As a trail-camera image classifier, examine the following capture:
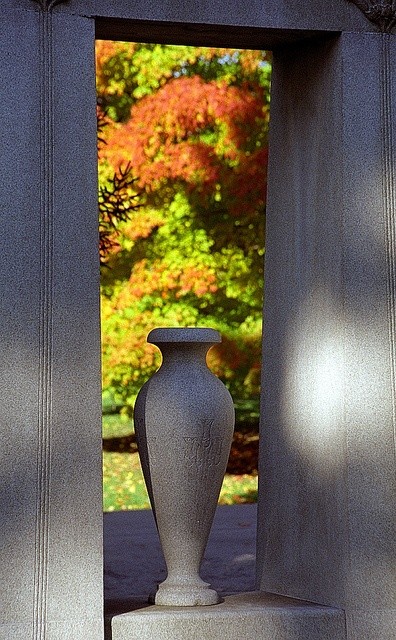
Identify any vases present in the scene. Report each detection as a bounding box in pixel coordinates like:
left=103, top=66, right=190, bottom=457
left=134, top=327, right=236, bottom=608
